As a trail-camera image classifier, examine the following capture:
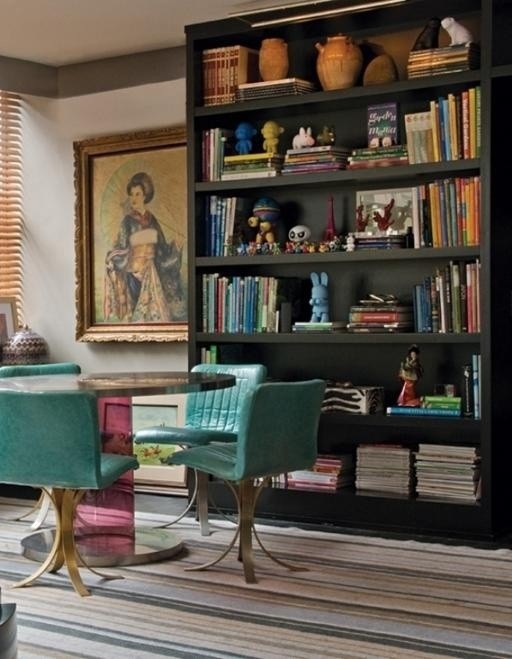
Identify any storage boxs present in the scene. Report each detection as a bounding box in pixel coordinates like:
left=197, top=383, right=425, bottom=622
left=320, top=379, right=387, bottom=417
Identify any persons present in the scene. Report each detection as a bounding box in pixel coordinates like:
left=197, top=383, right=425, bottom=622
left=396, top=345, right=424, bottom=407
left=98, top=171, right=183, bottom=326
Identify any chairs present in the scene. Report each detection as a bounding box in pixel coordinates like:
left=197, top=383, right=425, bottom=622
left=129, top=360, right=269, bottom=543
left=163, top=373, right=331, bottom=585
left=0, top=360, right=98, bottom=530
left=0, top=386, right=128, bottom=600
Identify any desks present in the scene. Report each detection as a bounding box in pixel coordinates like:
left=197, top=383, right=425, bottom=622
left=0, top=370, right=238, bottom=570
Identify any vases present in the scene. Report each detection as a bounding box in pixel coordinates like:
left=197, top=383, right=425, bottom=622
left=258, top=38, right=290, bottom=82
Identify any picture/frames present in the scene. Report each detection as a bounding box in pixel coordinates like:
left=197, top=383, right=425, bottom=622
left=95, top=393, right=188, bottom=489
left=0, top=295, right=22, bottom=354
left=65, top=120, right=187, bottom=345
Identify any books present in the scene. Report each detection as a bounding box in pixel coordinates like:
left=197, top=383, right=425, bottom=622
left=203, top=260, right=481, bottom=333
left=386, top=354, right=481, bottom=420
left=202, top=87, right=483, bottom=182
left=202, top=45, right=314, bottom=106
left=406, top=44, right=480, bottom=78
left=253, top=443, right=481, bottom=501
left=412, top=176, right=483, bottom=248
left=204, top=195, right=253, bottom=256
left=201, top=344, right=239, bottom=364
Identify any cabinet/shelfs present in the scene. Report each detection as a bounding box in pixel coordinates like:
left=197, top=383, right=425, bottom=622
left=183, top=0, right=511, bottom=550
